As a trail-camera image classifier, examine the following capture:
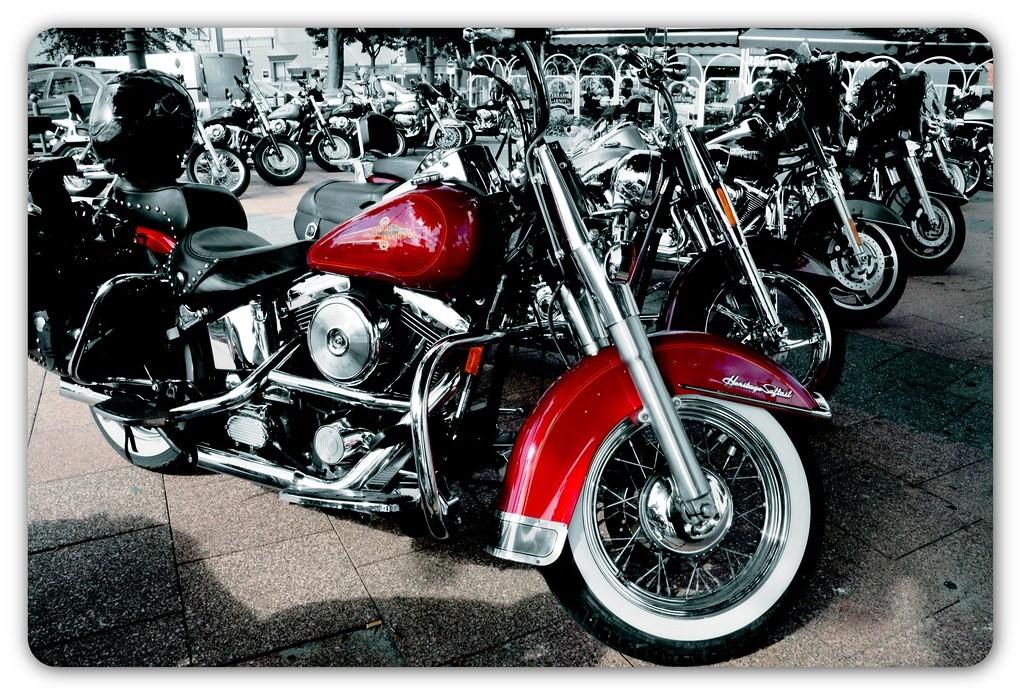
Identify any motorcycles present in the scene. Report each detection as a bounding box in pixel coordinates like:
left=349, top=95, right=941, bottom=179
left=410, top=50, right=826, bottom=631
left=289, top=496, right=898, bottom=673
left=27, top=27, right=994, bottom=669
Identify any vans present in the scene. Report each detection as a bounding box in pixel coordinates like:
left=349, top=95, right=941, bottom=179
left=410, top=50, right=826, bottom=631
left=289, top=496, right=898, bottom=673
left=61, top=54, right=250, bottom=122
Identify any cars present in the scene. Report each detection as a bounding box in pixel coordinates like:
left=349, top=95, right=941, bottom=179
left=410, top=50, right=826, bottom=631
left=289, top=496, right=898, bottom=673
left=29, top=67, right=122, bottom=121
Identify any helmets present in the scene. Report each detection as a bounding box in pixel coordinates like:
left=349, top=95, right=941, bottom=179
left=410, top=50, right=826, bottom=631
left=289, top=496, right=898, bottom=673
left=88, top=67, right=199, bottom=182
